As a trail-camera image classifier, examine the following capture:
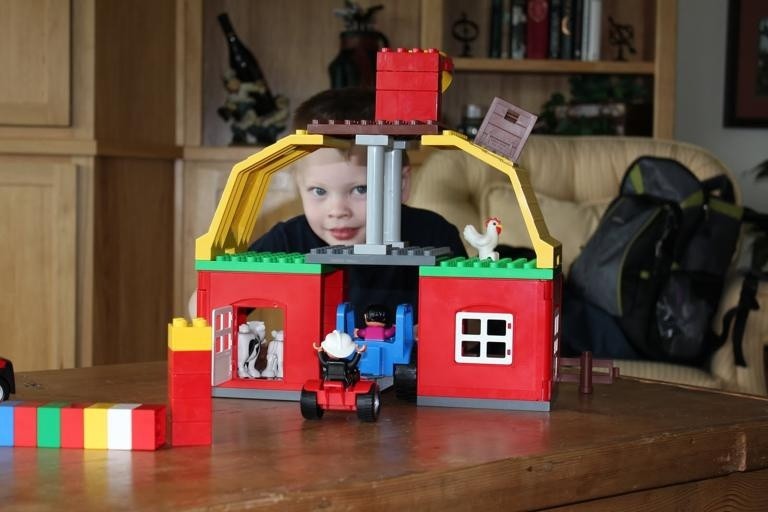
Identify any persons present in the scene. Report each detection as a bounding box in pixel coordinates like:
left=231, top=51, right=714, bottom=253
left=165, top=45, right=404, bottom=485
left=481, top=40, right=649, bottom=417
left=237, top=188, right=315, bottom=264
left=247, top=86, right=470, bottom=330
left=354, top=305, right=395, bottom=341
left=313, top=330, right=366, bottom=372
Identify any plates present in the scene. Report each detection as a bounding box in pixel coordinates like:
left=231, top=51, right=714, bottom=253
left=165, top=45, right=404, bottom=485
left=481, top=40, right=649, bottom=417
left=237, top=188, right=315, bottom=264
left=167, top=3, right=686, bottom=333
left=0, top=2, right=183, bottom=375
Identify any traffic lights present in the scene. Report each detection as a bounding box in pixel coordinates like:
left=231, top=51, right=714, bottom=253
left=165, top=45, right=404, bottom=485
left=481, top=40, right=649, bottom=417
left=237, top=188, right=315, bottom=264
left=465, top=180, right=618, bottom=287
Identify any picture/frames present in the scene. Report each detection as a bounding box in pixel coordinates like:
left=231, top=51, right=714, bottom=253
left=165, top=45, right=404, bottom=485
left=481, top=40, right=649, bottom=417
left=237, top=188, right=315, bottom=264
left=0, top=352, right=767, bottom=511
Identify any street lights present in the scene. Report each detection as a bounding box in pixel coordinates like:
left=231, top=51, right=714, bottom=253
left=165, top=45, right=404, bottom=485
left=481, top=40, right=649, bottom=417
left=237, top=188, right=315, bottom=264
left=559, top=155, right=767, bottom=370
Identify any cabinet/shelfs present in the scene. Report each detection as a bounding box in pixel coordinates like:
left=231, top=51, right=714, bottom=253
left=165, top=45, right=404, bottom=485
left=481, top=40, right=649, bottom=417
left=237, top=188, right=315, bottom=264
left=214, top=12, right=277, bottom=118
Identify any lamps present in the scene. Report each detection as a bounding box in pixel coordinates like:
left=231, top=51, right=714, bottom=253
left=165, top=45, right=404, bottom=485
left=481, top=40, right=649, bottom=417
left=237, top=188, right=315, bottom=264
left=397, top=132, right=766, bottom=401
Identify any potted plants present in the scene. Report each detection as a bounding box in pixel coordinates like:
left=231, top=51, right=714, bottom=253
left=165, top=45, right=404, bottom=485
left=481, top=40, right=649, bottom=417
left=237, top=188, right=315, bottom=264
left=722, top=0, right=767, bottom=133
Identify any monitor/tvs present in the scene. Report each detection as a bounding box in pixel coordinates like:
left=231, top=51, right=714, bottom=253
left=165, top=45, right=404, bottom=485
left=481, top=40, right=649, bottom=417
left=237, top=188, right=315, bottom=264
left=489, top=2, right=603, bottom=62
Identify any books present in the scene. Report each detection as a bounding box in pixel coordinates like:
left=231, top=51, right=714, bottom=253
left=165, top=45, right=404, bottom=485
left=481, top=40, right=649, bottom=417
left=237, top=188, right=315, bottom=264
left=489, top=2, right=603, bottom=62
